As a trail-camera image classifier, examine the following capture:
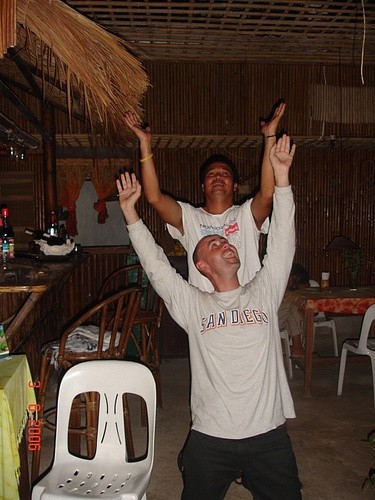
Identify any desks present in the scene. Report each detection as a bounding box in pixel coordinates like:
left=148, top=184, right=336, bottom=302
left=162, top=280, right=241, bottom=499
left=0, top=251, right=90, bottom=354
left=0, top=355, right=39, bottom=500
left=283, top=284, right=375, bottom=397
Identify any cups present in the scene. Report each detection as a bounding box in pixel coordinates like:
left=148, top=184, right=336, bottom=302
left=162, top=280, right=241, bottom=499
left=320, top=279, right=331, bottom=292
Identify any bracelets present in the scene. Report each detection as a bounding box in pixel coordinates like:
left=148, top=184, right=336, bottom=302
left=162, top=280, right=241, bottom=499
left=140, top=153, right=152, bottom=162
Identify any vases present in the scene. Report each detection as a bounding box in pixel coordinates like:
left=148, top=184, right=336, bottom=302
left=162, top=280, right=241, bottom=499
left=349, top=268, right=358, bottom=289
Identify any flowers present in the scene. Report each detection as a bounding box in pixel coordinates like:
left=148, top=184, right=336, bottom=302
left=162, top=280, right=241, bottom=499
left=338, top=242, right=372, bottom=273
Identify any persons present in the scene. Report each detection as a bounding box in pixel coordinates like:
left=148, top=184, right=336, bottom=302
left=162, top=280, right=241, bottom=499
left=124, top=104, right=287, bottom=294
left=116, top=134, right=304, bottom=500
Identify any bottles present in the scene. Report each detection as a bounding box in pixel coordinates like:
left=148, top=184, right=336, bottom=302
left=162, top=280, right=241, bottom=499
left=48, top=211, right=59, bottom=237
left=0, top=208, right=15, bottom=264
left=24, top=228, right=50, bottom=241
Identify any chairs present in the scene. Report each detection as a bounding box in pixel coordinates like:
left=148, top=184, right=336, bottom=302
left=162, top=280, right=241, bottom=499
left=278, top=278, right=375, bottom=396
left=27, top=265, right=167, bottom=500
